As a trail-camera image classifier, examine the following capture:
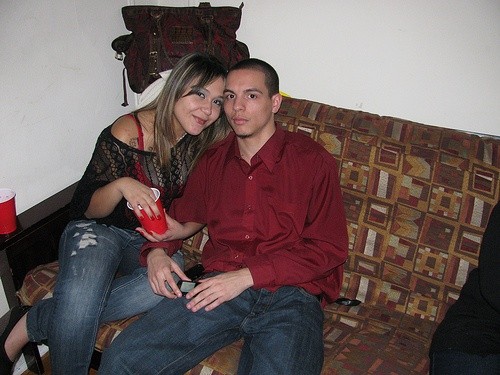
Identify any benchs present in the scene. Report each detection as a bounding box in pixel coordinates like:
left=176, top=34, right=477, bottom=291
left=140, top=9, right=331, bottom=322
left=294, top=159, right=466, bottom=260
left=1, top=94, right=499, bottom=375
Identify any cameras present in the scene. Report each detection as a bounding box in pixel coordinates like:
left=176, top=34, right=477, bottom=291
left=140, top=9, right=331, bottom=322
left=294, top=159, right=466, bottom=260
left=173, top=280, right=203, bottom=297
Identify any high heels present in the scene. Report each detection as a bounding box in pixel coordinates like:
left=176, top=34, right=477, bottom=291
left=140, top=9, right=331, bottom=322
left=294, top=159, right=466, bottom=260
left=0, top=304, right=31, bottom=375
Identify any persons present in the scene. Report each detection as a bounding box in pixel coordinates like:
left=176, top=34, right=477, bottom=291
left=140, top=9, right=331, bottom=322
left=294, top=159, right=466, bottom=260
left=429, top=198, right=500, bottom=375
left=95, top=59, right=349, bottom=375
left=1, top=52, right=234, bottom=375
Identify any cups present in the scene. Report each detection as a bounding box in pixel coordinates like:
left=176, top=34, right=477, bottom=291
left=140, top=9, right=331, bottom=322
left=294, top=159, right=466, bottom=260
left=127, top=188, right=168, bottom=235
left=0, top=188, right=16, bottom=234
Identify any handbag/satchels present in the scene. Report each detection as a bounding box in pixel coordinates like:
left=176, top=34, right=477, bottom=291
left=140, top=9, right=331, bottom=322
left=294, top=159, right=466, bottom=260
left=111, top=2, right=250, bottom=106
